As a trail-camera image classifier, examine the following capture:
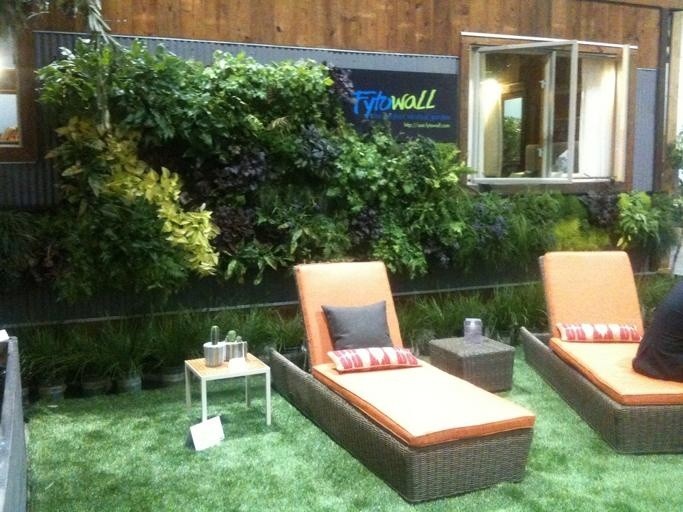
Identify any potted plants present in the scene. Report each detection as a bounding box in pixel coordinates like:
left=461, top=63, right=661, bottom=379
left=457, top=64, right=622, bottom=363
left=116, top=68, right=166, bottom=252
left=157, top=303, right=201, bottom=385
left=203, top=326, right=223, bottom=367
left=70, top=324, right=111, bottom=397
left=19, top=320, right=70, bottom=402
left=112, top=306, right=163, bottom=397
left=225, top=330, right=247, bottom=361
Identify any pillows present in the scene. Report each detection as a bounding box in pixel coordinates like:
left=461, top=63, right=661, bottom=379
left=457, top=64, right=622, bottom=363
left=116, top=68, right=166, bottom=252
left=321, top=300, right=394, bottom=351
left=324, top=346, right=422, bottom=375
left=554, top=320, right=643, bottom=342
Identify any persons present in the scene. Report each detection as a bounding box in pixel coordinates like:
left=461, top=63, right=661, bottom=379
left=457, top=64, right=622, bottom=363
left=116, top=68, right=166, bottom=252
left=634, top=271, right=679, bottom=379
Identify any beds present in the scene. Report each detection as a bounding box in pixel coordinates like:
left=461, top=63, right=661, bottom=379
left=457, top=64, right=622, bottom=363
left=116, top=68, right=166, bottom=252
left=269, top=261, right=536, bottom=503
left=519, top=251, right=682, bottom=454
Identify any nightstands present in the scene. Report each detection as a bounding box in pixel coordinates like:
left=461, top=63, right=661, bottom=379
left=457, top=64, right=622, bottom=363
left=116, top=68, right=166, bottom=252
left=429, top=336, right=515, bottom=393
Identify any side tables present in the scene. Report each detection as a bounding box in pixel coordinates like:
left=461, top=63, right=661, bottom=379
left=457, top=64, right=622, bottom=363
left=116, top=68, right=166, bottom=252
left=185, top=353, right=272, bottom=425
